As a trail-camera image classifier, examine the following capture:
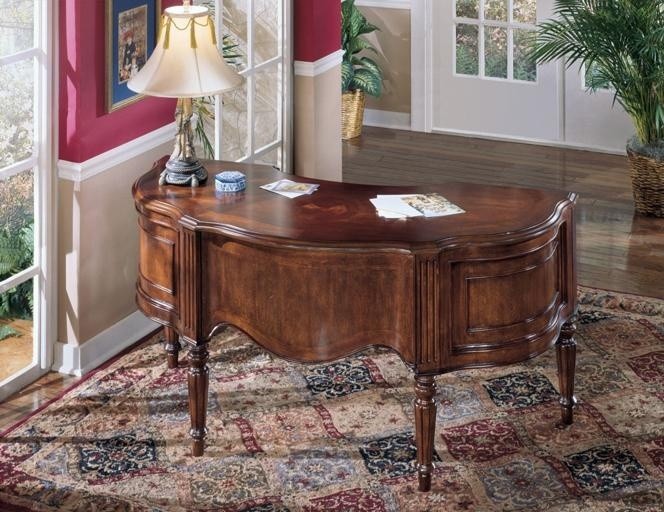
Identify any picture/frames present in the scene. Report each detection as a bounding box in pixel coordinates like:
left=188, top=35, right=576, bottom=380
left=103, top=0, right=162, bottom=116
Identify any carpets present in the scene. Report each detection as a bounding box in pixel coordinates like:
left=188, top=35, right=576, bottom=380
left=0, top=284, right=664, bottom=512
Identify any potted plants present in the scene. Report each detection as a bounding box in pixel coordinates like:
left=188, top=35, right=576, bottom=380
left=341, top=1, right=385, bottom=142
left=519, top=1, right=663, bottom=220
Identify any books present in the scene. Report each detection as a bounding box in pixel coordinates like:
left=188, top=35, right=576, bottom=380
left=256, top=175, right=321, bottom=202
left=368, top=190, right=466, bottom=223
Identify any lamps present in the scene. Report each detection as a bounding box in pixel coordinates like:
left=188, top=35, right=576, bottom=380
left=126, top=4, right=249, bottom=191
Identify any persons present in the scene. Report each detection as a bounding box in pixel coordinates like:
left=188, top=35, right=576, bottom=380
left=121, top=27, right=139, bottom=71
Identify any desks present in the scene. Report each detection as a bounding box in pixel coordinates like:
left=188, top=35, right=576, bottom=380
left=131, top=153, right=579, bottom=495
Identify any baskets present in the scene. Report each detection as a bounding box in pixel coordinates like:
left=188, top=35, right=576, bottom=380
left=342, top=89, right=365, bottom=141
left=627, top=136, right=663, bottom=216
